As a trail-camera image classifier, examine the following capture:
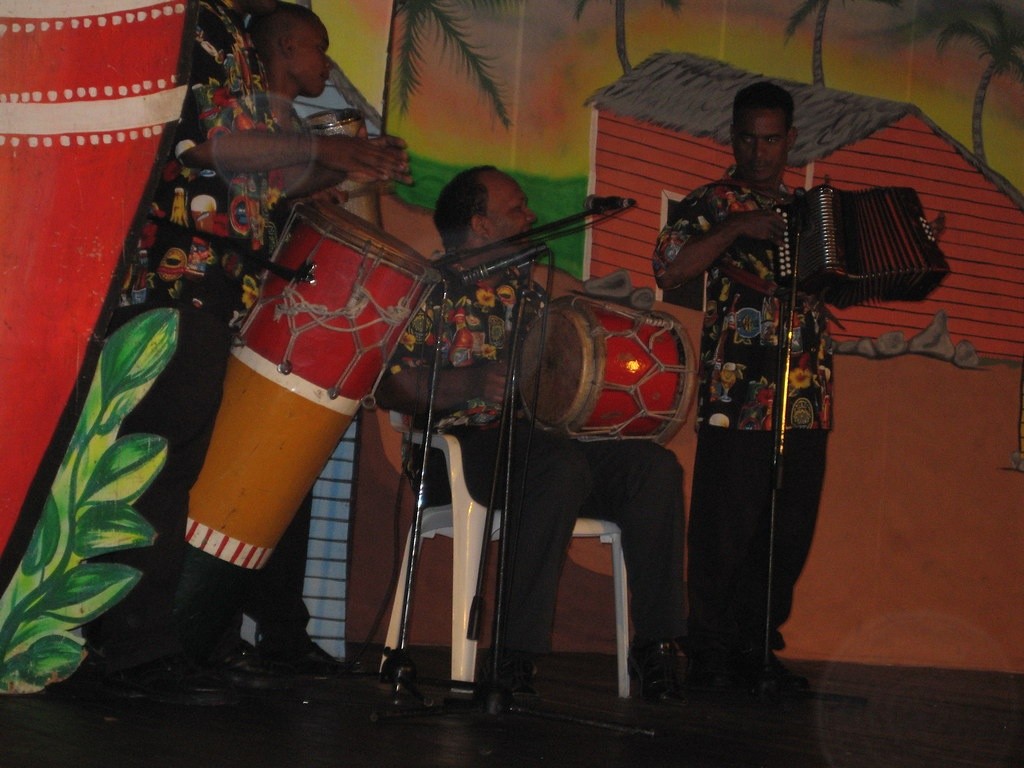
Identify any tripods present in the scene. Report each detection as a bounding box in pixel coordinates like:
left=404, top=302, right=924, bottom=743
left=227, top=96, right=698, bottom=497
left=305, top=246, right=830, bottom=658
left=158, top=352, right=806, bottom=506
left=369, top=248, right=656, bottom=740
left=321, top=210, right=606, bottom=709
left=681, top=187, right=869, bottom=713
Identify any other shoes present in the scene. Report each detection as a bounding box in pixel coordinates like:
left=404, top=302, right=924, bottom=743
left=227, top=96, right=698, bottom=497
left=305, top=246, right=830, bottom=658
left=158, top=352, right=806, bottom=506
left=256, top=635, right=336, bottom=675
left=688, top=657, right=778, bottom=705
left=626, top=635, right=684, bottom=701
left=487, top=644, right=537, bottom=702
left=198, top=643, right=294, bottom=689
left=726, top=650, right=810, bottom=693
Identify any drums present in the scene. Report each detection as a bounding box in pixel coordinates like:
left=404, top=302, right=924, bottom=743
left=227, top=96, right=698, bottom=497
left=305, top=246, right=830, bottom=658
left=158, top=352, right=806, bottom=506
left=515, top=289, right=701, bottom=447
left=183, top=189, right=443, bottom=575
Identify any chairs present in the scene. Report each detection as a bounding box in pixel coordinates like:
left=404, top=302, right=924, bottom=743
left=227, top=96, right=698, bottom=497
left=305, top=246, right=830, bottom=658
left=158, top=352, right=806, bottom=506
left=379, top=410, right=631, bottom=701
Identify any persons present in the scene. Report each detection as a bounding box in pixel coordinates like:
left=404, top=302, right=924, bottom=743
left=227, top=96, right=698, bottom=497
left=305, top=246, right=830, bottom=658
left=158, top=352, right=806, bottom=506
left=652, top=81, right=946, bottom=703
left=80, top=2, right=414, bottom=704
left=249, top=2, right=361, bottom=681
left=375, top=163, right=690, bottom=700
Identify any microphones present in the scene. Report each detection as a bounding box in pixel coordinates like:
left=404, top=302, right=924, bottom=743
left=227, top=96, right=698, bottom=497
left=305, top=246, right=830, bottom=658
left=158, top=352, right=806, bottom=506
left=583, top=194, right=636, bottom=210
left=457, top=241, right=548, bottom=287
